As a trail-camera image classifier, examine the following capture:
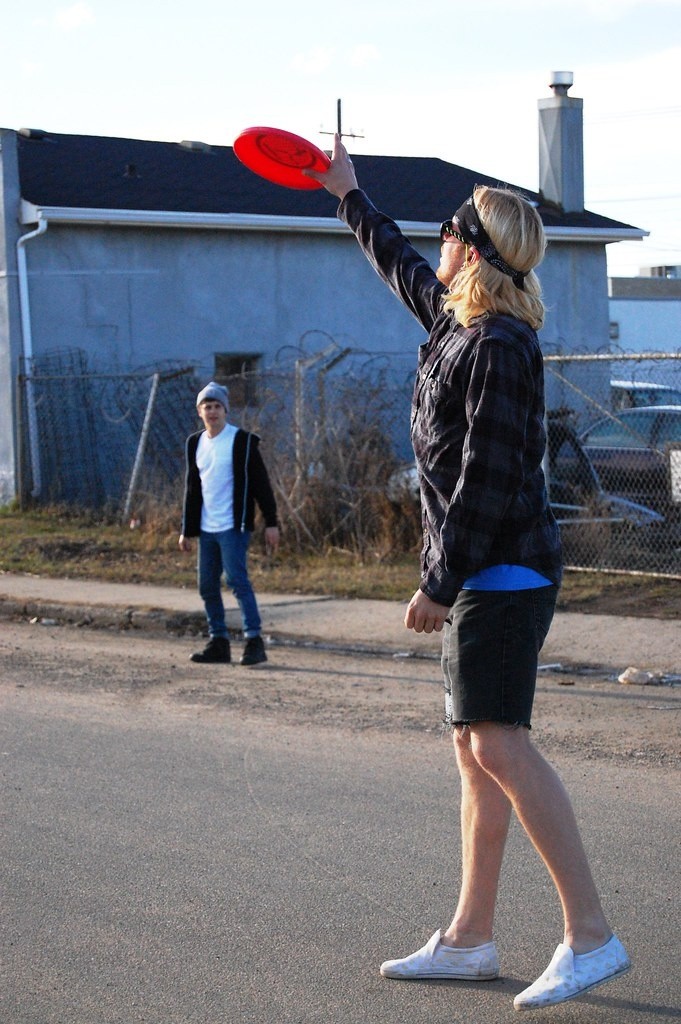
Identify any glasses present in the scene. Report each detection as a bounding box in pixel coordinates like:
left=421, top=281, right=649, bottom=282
left=438, top=219, right=472, bottom=248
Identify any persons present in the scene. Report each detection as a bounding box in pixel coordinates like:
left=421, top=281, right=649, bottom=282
left=302, top=133, right=633, bottom=1011
left=179, top=382, right=279, bottom=666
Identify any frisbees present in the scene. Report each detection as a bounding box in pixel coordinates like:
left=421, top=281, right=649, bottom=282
left=233, top=126, right=331, bottom=191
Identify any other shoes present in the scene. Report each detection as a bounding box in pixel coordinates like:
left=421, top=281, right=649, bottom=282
left=512, top=933, right=634, bottom=1012
left=190, top=638, right=230, bottom=662
left=379, top=926, right=499, bottom=980
left=240, top=637, right=268, bottom=664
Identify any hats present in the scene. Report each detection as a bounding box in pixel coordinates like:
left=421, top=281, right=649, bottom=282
left=197, top=381, right=231, bottom=413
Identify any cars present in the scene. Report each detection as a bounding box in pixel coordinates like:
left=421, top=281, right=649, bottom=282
left=556, top=406, right=681, bottom=502
